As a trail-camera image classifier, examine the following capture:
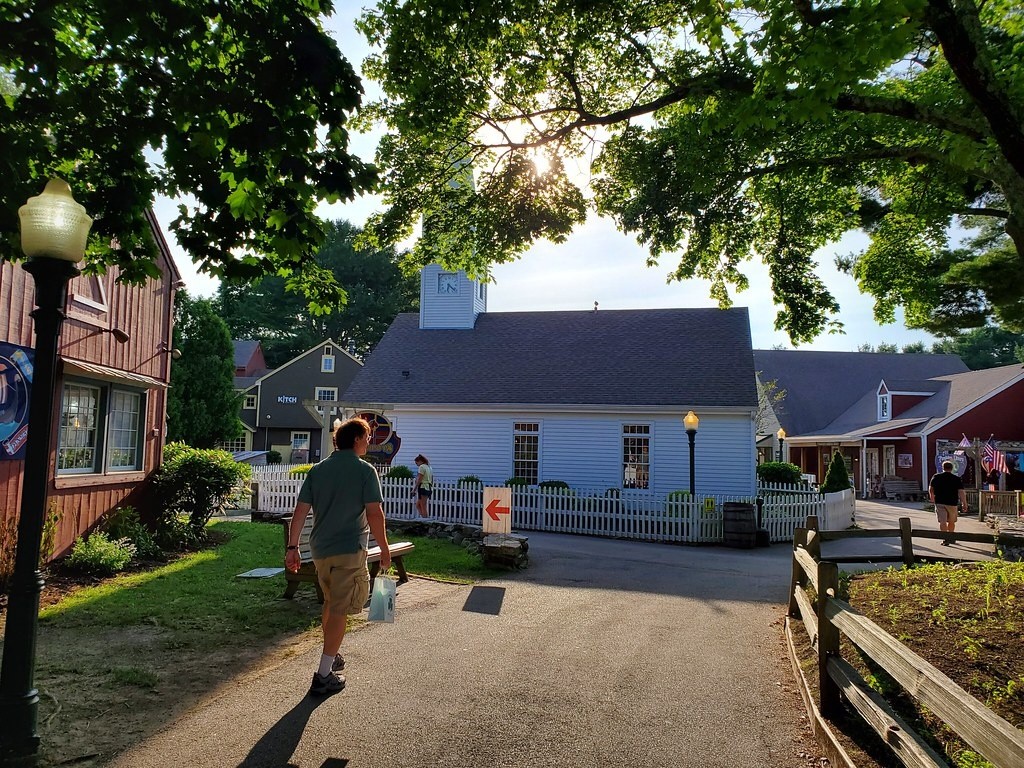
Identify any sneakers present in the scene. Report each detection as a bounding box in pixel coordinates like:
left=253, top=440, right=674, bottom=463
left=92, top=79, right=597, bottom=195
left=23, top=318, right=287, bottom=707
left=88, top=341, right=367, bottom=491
left=332, top=653, right=345, bottom=671
left=310, top=670, right=346, bottom=695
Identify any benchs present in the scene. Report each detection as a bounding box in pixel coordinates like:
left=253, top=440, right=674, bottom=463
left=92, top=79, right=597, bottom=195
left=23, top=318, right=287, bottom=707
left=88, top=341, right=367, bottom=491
left=281, top=515, right=415, bottom=604
left=874, top=479, right=928, bottom=501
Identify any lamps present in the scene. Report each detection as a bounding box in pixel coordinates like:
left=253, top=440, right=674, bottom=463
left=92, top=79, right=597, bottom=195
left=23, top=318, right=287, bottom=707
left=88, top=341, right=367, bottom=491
left=162, top=347, right=182, bottom=358
left=402, top=371, right=409, bottom=379
left=99, top=327, right=130, bottom=344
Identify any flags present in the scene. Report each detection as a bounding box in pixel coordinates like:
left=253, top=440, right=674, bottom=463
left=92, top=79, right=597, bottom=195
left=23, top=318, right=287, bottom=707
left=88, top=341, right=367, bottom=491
left=954, top=437, right=971, bottom=455
left=984, top=436, right=1010, bottom=474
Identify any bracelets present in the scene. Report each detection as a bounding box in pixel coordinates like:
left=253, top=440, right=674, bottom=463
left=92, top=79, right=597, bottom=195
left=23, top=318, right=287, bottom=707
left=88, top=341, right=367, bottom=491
left=286, top=545, right=299, bottom=550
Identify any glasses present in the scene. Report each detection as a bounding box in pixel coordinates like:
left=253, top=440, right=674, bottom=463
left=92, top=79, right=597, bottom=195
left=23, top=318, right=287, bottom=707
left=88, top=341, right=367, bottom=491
left=362, top=436, right=371, bottom=442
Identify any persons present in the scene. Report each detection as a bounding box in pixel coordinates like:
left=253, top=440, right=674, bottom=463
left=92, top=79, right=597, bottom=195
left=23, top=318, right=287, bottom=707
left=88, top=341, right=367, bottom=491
left=986, top=469, right=999, bottom=484
left=411, top=454, right=433, bottom=518
left=929, top=461, right=968, bottom=546
left=285, top=418, right=391, bottom=690
left=637, top=445, right=648, bottom=486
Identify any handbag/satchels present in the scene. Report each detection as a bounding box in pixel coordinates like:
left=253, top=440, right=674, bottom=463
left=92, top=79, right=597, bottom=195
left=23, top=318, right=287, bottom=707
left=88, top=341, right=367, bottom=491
left=367, top=566, right=396, bottom=623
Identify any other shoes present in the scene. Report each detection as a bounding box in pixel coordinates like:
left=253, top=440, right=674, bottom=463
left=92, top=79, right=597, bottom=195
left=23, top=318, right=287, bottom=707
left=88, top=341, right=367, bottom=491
left=941, top=538, right=955, bottom=546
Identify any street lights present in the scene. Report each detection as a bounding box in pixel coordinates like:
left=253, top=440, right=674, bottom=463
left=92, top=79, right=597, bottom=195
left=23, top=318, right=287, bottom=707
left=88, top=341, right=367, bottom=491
left=0, top=172, right=95, bottom=762
left=777, top=427, right=787, bottom=463
left=682, top=410, right=700, bottom=503
